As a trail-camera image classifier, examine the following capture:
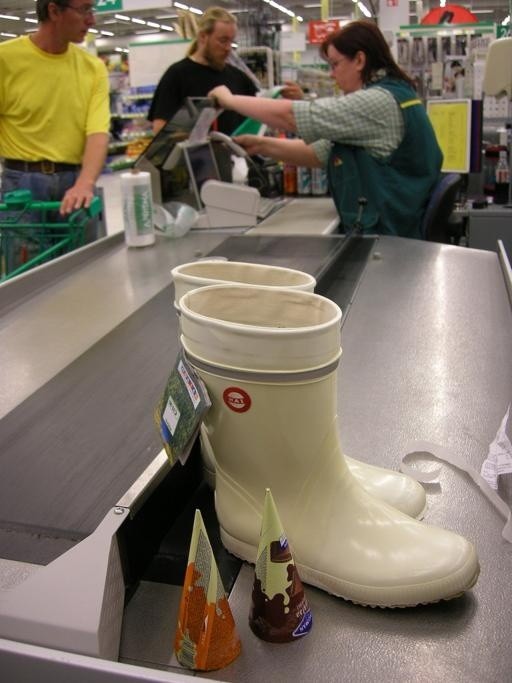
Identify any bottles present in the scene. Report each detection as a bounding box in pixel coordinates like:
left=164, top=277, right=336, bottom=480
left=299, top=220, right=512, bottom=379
left=493, top=151, right=510, bottom=205
left=232, top=157, right=250, bottom=186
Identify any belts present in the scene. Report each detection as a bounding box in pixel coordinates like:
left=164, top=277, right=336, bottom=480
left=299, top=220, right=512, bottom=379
left=4, top=158, right=82, bottom=175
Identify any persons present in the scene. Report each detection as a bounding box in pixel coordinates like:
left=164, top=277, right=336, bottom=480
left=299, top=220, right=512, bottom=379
left=0, top=0, right=112, bottom=283
left=206, top=18, right=442, bottom=241
left=147, top=5, right=306, bottom=165
left=445, top=61, right=466, bottom=93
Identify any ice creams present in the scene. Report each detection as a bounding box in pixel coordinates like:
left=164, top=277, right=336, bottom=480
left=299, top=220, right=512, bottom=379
left=248, top=488, right=312, bottom=643
left=175, top=508, right=241, bottom=671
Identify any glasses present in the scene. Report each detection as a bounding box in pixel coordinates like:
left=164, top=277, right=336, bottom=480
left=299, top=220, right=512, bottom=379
left=67, top=3, right=93, bottom=13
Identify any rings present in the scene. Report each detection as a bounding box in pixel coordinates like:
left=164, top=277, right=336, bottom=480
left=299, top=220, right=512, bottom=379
left=289, top=91, right=293, bottom=97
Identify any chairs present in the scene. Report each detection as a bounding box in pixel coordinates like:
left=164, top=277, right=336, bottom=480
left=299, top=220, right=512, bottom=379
left=421, top=172, right=463, bottom=243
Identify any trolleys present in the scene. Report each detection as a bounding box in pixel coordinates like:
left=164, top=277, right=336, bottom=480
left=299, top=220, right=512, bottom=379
left=1, top=187, right=104, bottom=282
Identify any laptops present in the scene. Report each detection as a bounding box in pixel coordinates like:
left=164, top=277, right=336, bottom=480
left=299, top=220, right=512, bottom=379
left=182, top=141, right=276, bottom=218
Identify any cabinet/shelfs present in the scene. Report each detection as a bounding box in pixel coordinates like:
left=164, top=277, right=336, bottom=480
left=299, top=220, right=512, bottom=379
left=95, top=38, right=196, bottom=173
